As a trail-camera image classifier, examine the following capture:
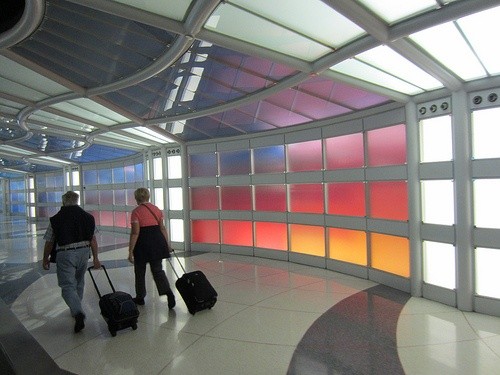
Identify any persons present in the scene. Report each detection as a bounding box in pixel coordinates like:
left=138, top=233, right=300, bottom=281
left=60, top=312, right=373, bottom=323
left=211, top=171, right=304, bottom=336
left=42, top=190, right=101, bottom=334
left=127, top=187, right=176, bottom=310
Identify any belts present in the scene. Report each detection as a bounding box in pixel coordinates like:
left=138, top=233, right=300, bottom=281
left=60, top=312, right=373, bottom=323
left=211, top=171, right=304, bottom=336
left=56, top=244, right=91, bottom=253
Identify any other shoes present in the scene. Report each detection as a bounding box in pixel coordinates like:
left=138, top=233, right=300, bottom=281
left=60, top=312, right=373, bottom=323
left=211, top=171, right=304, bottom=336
left=74, top=313, right=85, bottom=334
left=169, top=292, right=175, bottom=309
left=132, top=297, right=145, bottom=305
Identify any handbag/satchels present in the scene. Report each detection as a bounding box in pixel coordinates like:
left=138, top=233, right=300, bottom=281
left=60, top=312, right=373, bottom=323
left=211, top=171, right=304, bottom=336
left=160, top=231, right=170, bottom=259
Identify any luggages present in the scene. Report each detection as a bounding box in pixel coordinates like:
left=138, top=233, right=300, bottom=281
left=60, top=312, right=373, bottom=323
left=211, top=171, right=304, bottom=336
left=167, top=249, right=218, bottom=316
left=88, top=265, right=139, bottom=336
